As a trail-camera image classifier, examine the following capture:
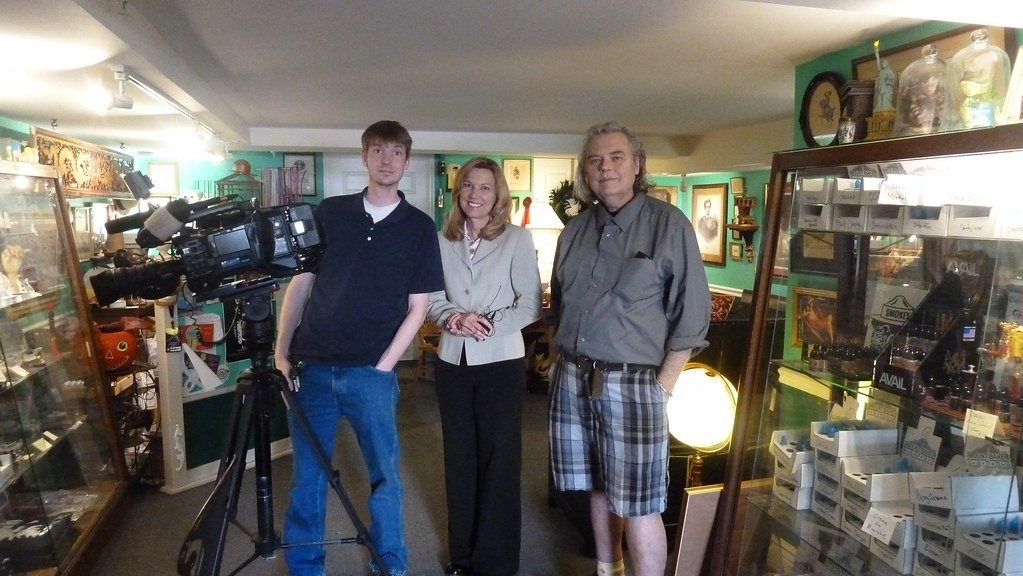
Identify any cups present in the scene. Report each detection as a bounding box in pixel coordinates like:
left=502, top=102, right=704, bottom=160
left=838, top=117, right=856, bottom=144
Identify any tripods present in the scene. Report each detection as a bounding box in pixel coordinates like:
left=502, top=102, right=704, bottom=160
left=177, top=274, right=393, bottom=576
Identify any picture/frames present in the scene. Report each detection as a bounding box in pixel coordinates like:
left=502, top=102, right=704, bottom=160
left=730, top=177, right=746, bottom=195
left=791, top=286, right=837, bottom=349
left=850, top=23, right=1017, bottom=107
left=501, top=158, right=532, bottom=193
left=282, top=152, right=317, bottom=196
left=647, top=186, right=677, bottom=205
left=790, top=168, right=847, bottom=279
left=670, top=477, right=774, bottom=576
left=800, top=71, right=848, bottom=147
left=507, top=197, right=520, bottom=223
left=729, top=242, right=743, bottom=259
left=146, top=162, right=180, bottom=195
left=446, top=174, right=457, bottom=192
left=692, top=183, right=728, bottom=267
left=731, top=219, right=742, bottom=240
left=765, top=182, right=792, bottom=281
left=28, top=124, right=135, bottom=199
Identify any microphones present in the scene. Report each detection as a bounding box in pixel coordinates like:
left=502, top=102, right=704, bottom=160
left=105, top=194, right=239, bottom=234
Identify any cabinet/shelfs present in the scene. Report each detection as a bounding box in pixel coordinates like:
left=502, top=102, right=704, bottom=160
left=0, top=158, right=150, bottom=576
left=707, top=122, right=1023, bottom=576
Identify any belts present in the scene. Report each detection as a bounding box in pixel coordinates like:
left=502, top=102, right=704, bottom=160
left=562, top=352, right=661, bottom=370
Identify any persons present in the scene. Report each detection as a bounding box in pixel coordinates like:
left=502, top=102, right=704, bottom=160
left=873, top=50, right=897, bottom=111
left=548, top=122, right=712, bottom=576
left=274, top=122, right=446, bottom=576
left=425, top=157, right=543, bottom=575
left=0, top=236, right=37, bottom=298
left=901, top=77, right=944, bottom=135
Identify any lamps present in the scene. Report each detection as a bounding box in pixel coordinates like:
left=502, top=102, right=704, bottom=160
left=103, top=92, right=134, bottom=111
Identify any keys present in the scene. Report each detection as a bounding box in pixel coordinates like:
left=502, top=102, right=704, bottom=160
left=289, top=364, right=301, bottom=392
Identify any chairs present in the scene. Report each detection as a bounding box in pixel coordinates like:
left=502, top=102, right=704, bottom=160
left=417, top=316, right=441, bottom=381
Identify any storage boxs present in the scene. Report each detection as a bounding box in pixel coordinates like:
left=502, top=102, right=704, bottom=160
left=953, top=511, right=1023, bottom=574
left=915, top=527, right=956, bottom=571
left=902, top=205, right=951, bottom=237
left=768, top=395, right=943, bottom=575
left=908, top=472, right=1019, bottom=509
left=947, top=205, right=1023, bottom=240
left=859, top=177, right=886, bottom=206
left=797, top=204, right=832, bottom=230
left=955, top=552, right=1023, bottom=576
left=878, top=162, right=913, bottom=177
left=878, top=180, right=921, bottom=207
left=798, top=175, right=835, bottom=206
left=847, top=164, right=882, bottom=179
left=922, top=172, right=984, bottom=205
left=832, top=204, right=868, bottom=233
left=912, top=502, right=1020, bottom=539
left=866, top=205, right=904, bottom=235
left=832, top=177, right=861, bottom=204
left=911, top=551, right=955, bottom=576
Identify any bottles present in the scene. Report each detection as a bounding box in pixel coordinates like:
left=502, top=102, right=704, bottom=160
left=800, top=339, right=879, bottom=377
left=887, top=309, right=957, bottom=369
left=937, top=372, right=1023, bottom=431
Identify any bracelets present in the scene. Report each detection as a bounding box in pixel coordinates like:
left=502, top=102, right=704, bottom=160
left=657, top=380, right=672, bottom=397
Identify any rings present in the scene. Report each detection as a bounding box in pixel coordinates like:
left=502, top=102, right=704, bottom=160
left=475, top=330, right=478, bottom=333
